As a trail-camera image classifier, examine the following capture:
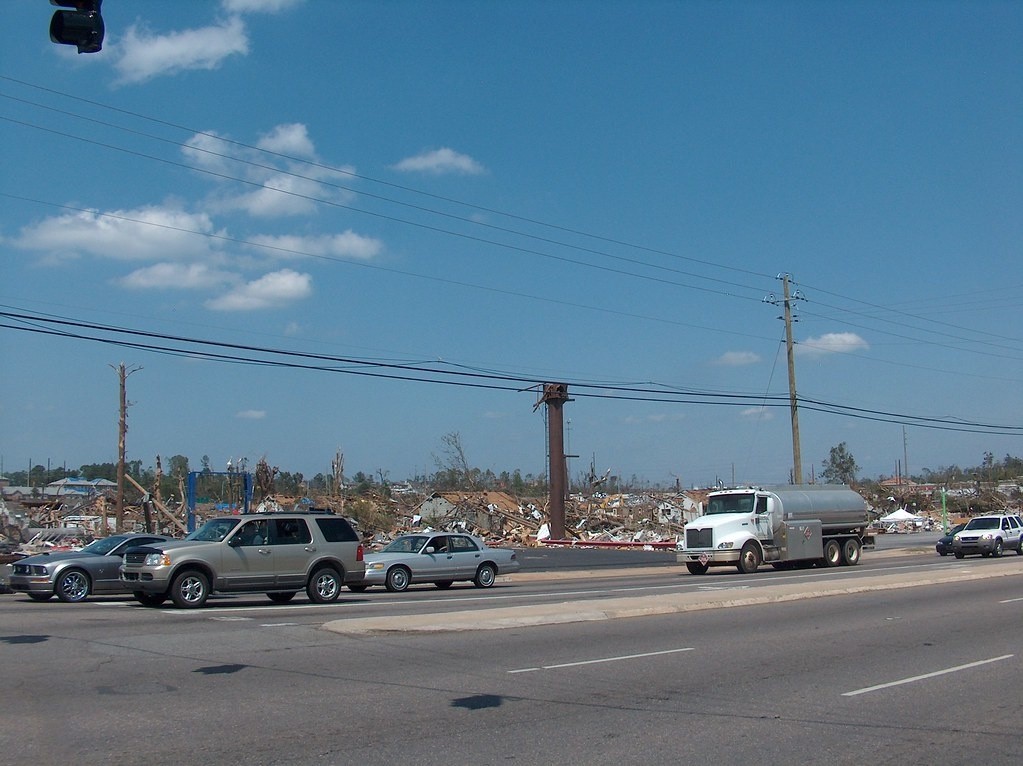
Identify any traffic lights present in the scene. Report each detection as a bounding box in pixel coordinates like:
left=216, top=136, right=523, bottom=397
left=49, top=1, right=105, bottom=55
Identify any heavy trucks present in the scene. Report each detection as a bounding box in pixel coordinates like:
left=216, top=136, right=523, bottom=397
left=675, top=483, right=869, bottom=576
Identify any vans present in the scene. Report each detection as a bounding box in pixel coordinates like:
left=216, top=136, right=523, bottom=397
left=118, top=508, right=367, bottom=608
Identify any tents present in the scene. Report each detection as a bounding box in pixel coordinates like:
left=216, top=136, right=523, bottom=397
left=880, top=508, right=923, bottom=534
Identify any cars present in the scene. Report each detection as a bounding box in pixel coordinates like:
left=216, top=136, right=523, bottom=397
left=936, top=521, right=966, bottom=556
left=8, top=532, right=178, bottom=603
left=952, top=513, right=1023, bottom=560
left=349, top=531, right=520, bottom=593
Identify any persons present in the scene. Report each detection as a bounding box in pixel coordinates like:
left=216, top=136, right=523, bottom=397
left=244, top=523, right=263, bottom=545
left=929, top=520, right=934, bottom=531
